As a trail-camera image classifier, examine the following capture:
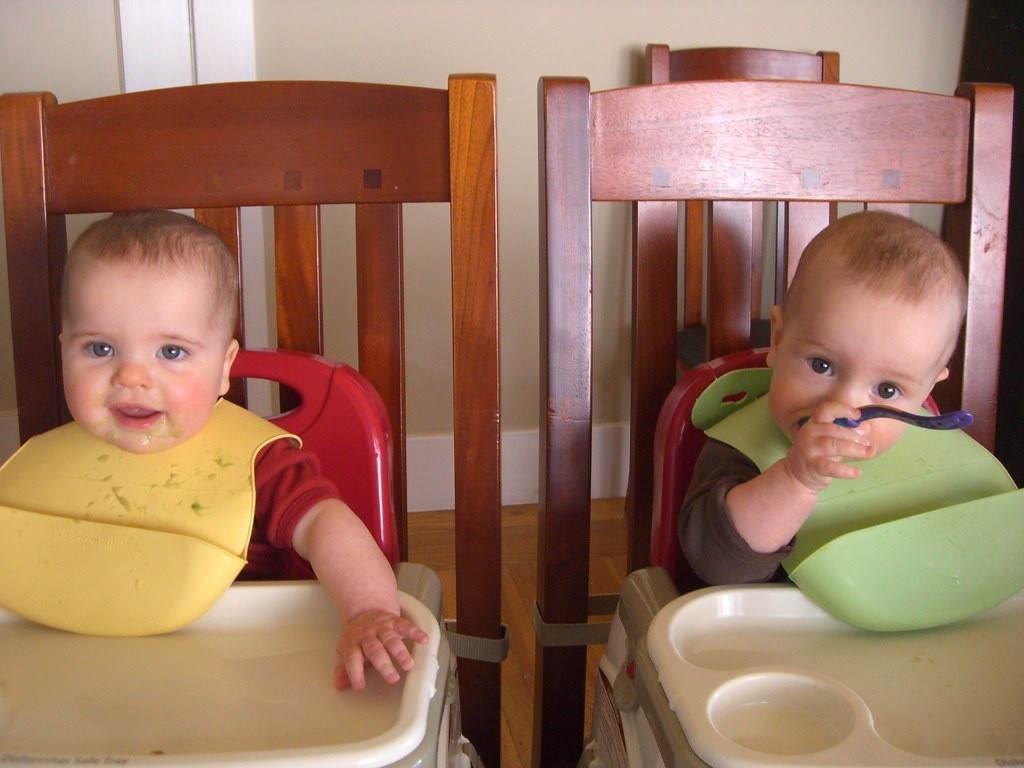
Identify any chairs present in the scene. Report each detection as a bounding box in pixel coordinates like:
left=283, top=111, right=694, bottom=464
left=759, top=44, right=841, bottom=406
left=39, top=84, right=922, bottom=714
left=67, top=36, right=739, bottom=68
left=0, top=74, right=509, bottom=768
left=536, top=43, right=1023, bottom=768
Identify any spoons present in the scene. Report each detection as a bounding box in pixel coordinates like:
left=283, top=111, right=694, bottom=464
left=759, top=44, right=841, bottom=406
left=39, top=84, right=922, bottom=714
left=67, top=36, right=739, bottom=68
left=799, top=404, right=974, bottom=430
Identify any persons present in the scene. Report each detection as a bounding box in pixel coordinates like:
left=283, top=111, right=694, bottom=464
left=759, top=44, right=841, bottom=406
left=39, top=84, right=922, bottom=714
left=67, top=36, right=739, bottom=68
left=675, top=210, right=969, bottom=585
left=0, top=205, right=430, bottom=692
